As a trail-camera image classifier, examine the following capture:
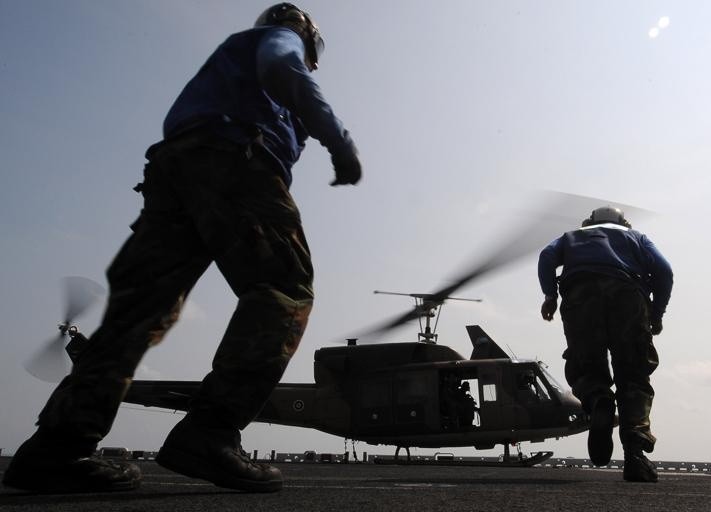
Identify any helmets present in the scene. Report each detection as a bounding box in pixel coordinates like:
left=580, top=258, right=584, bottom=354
left=254, top=2, right=325, bottom=72
left=591, top=205, right=622, bottom=223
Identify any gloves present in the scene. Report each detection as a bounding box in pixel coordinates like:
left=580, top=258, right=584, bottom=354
left=540, top=292, right=560, bottom=322
left=650, top=301, right=664, bottom=333
left=328, top=130, right=362, bottom=186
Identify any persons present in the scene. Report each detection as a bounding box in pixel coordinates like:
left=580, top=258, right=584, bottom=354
left=0, top=0, right=366, bottom=495
left=532, top=201, right=676, bottom=483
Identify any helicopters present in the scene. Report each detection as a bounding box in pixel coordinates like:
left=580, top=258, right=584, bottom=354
left=25, top=193, right=651, bottom=466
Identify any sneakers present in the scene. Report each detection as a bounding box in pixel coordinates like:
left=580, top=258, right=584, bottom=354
left=156, top=430, right=284, bottom=492
left=587, top=397, right=613, bottom=465
left=625, top=460, right=660, bottom=483
left=6, top=437, right=142, bottom=491
